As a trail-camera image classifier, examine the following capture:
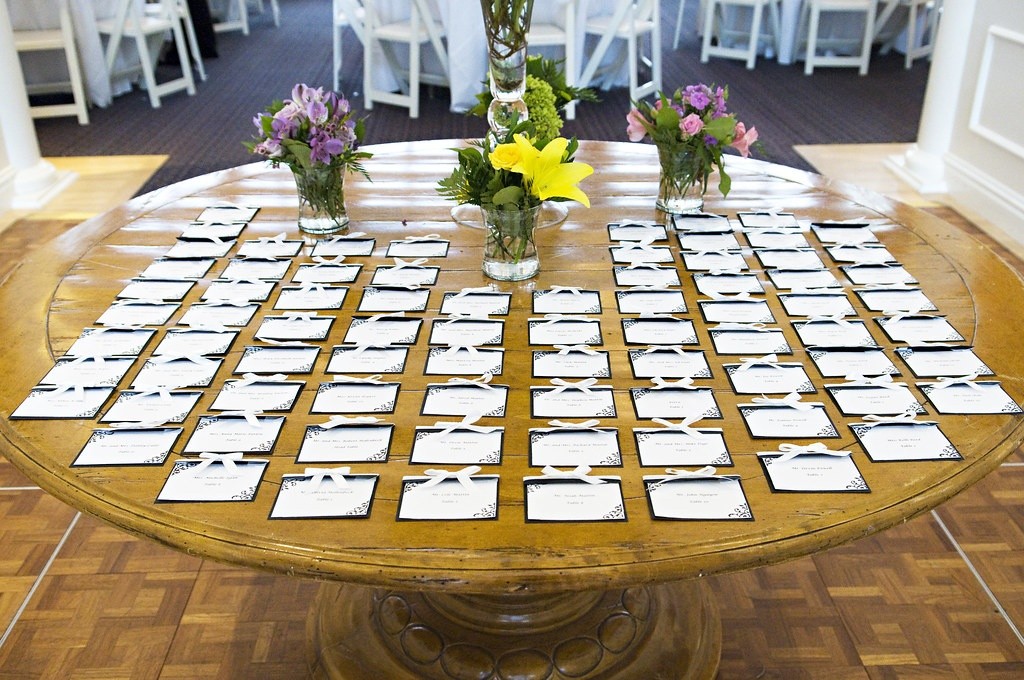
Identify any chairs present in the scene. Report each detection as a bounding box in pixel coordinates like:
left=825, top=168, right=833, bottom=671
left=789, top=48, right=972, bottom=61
left=6, top=0, right=942, bottom=127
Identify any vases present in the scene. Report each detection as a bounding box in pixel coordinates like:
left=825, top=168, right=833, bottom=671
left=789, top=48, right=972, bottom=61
left=289, top=166, right=351, bottom=234
left=655, top=138, right=708, bottom=212
left=481, top=201, right=542, bottom=281
left=450, top=0, right=568, bottom=231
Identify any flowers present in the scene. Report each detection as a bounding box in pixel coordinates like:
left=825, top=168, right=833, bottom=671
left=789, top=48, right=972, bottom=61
left=241, top=82, right=374, bottom=226
left=468, top=52, right=602, bottom=161
left=626, top=81, right=769, bottom=204
left=433, top=129, right=594, bottom=262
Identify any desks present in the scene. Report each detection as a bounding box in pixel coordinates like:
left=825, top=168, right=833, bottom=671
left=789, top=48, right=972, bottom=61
left=0, top=0, right=173, bottom=107
left=699, top=0, right=921, bottom=60
left=0, top=139, right=1024, bottom=680
left=356, top=0, right=648, bottom=113
